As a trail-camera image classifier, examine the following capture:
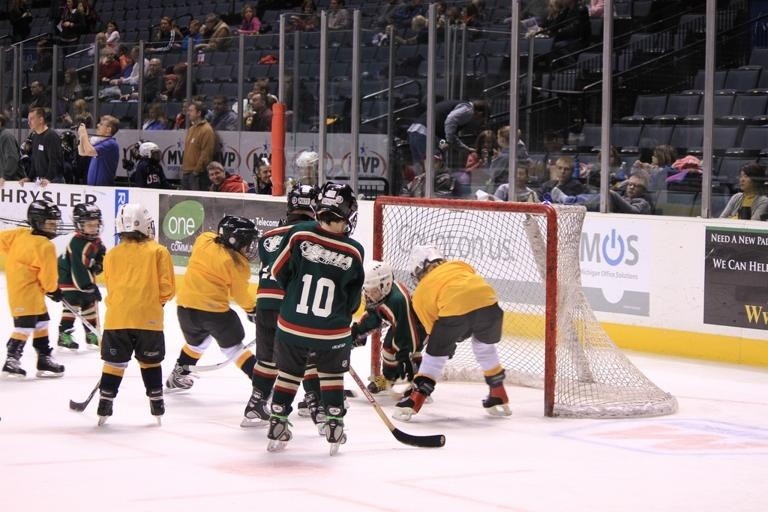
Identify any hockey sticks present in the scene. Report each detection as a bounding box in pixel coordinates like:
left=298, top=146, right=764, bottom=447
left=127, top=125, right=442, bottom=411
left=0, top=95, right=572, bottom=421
left=183, top=337, right=257, bottom=372
left=348, top=364, right=445, bottom=447
left=69, top=378, right=101, bottom=411
left=344, top=389, right=358, bottom=396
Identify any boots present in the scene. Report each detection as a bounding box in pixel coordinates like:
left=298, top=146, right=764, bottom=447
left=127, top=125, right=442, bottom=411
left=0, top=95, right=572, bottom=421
left=244, top=369, right=508, bottom=443
left=2, top=326, right=194, bottom=416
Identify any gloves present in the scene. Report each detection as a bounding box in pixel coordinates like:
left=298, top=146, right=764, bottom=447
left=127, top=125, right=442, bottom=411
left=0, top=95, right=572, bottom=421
left=84, top=285, right=102, bottom=300
left=47, top=288, right=65, bottom=302
left=89, top=264, right=102, bottom=274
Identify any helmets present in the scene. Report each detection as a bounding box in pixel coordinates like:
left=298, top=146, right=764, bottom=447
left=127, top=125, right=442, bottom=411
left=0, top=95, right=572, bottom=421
left=217, top=151, right=445, bottom=310
left=138, top=142, right=162, bottom=162
left=28, top=200, right=155, bottom=241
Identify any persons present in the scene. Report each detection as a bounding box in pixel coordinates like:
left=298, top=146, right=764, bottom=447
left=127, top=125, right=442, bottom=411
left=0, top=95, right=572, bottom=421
left=168, top=214, right=262, bottom=388
left=0, top=199, right=65, bottom=375
left=1, top=1, right=767, bottom=221
left=97, top=202, right=177, bottom=418
left=244, top=180, right=324, bottom=425
left=266, top=181, right=365, bottom=444
left=51, top=203, right=106, bottom=349
left=351, top=250, right=428, bottom=394
left=394, top=244, right=511, bottom=415
